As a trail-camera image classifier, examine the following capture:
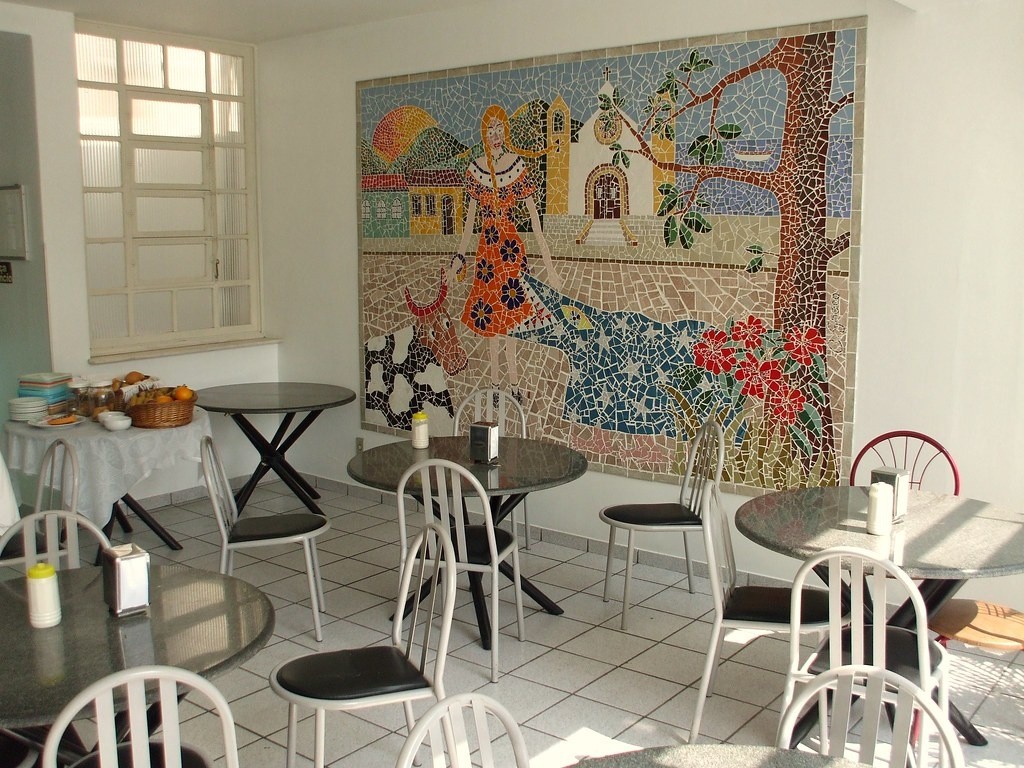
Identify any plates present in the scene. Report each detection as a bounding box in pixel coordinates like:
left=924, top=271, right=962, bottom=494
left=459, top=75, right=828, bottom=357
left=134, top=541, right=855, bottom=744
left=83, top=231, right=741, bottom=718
left=17, top=371, right=73, bottom=413
left=8, top=396, right=49, bottom=422
left=27, top=413, right=87, bottom=430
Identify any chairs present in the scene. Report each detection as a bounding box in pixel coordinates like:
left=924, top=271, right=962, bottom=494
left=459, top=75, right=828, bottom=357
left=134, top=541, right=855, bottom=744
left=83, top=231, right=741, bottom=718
left=777, top=665, right=964, bottom=768
left=451, top=388, right=530, bottom=551
left=201, top=434, right=331, bottom=642
left=911, top=598, right=1024, bottom=746
left=398, top=458, right=524, bottom=683
left=688, top=480, right=853, bottom=744
left=775, top=545, right=950, bottom=768
left=0, top=438, right=111, bottom=569
left=270, top=524, right=456, bottom=768
left=395, top=692, right=531, bottom=768
left=41, top=665, right=240, bottom=768
left=848, top=431, right=960, bottom=495
left=599, top=421, right=725, bottom=629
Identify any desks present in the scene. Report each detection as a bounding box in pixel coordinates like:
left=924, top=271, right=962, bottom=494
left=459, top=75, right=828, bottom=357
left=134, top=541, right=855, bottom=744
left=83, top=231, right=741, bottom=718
left=195, top=383, right=357, bottom=517
left=735, top=485, right=1024, bottom=750
left=346, top=436, right=589, bottom=651
left=563, top=744, right=873, bottom=768
left=3, top=406, right=223, bottom=566
left=0, top=564, right=276, bottom=764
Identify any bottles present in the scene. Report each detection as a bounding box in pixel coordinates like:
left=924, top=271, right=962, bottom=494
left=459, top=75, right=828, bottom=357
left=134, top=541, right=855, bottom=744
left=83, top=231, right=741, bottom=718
left=866, top=481, right=893, bottom=537
left=25, top=562, right=62, bottom=628
left=410, top=411, right=430, bottom=450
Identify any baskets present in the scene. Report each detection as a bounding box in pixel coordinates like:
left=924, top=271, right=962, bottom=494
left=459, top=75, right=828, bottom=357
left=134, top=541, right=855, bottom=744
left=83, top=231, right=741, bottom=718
left=125, top=387, right=198, bottom=429
left=72, top=375, right=158, bottom=416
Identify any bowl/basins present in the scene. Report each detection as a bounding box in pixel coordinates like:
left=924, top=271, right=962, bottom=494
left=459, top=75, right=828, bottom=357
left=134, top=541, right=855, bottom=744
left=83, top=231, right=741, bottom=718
left=98, top=410, right=124, bottom=426
left=107, top=416, right=133, bottom=432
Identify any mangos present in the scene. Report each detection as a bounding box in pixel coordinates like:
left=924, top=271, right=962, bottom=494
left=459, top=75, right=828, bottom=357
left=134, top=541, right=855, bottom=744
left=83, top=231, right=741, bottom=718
left=176, top=387, right=192, bottom=401
left=154, top=396, right=172, bottom=402
left=125, top=372, right=143, bottom=384
